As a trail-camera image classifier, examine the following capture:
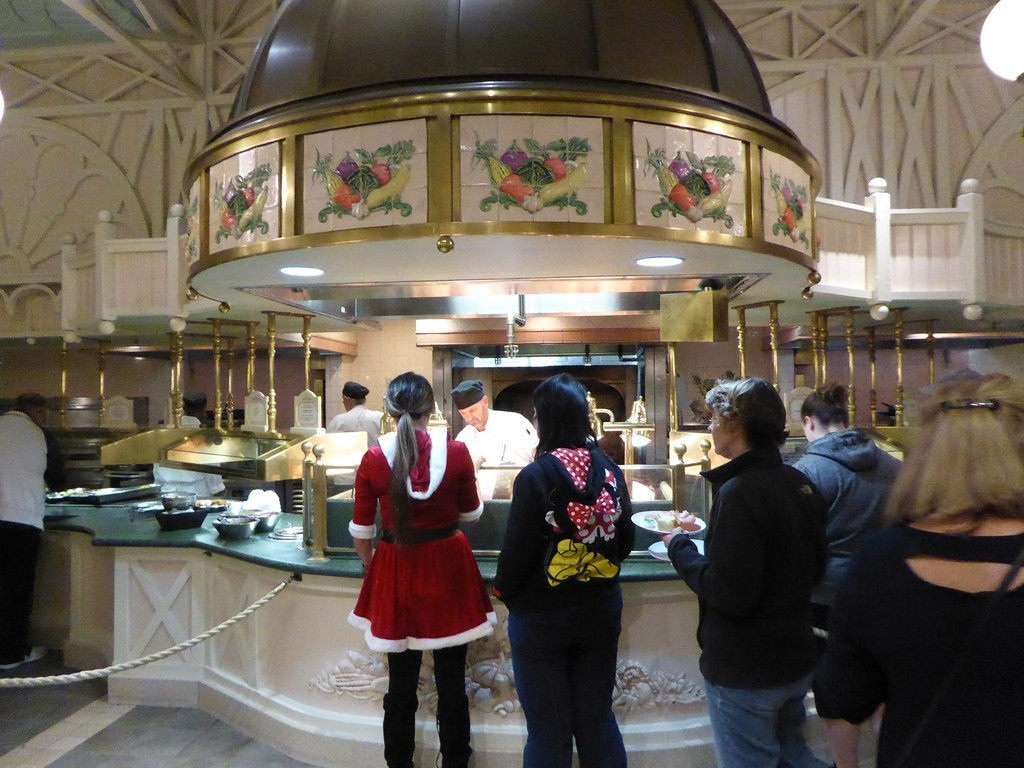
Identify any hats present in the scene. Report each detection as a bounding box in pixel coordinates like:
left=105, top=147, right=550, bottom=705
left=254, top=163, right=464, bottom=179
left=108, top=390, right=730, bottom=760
left=183, top=393, right=206, bottom=405
left=450, top=380, right=484, bottom=410
left=342, top=381, right=369, bottom=399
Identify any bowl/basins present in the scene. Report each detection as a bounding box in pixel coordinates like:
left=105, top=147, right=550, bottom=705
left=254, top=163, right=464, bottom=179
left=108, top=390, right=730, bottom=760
left=219, top=508, right=283, bottom=532
left=151, top=509, right=210, bottom=530
left=160, top=492, right=198, bottom=511
left=211, top=518, right=260, bottom=539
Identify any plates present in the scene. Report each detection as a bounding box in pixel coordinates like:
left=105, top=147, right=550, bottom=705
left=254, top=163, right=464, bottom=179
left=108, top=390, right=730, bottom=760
left=195, top=499, right=231, bottom=513
left=630, top=511, right=707, bottom=534
left=647, top=539, right=705, bottom=562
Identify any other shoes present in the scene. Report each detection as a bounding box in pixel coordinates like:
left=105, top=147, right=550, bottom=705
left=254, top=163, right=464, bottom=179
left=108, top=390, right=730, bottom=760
left=0, top=645, right=48, bottom=669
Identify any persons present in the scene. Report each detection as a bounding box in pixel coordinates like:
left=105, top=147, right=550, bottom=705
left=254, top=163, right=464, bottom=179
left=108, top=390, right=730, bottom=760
left=326, top=381, right=385, bottom=448
left=661, top=376, right=836, bottom=768
left=450, top=380, right=540, bottom=466
left=812, top=372, right=1024, bottom=768
left=346, top=372, right=499, bottom=768
left=493, top=372, right=636, bottom=768
left=0, top=392, right=67, bottom=670
left=180, top=390, right=208, bottom=424
left=791, top=383, right=903, bottom=768
